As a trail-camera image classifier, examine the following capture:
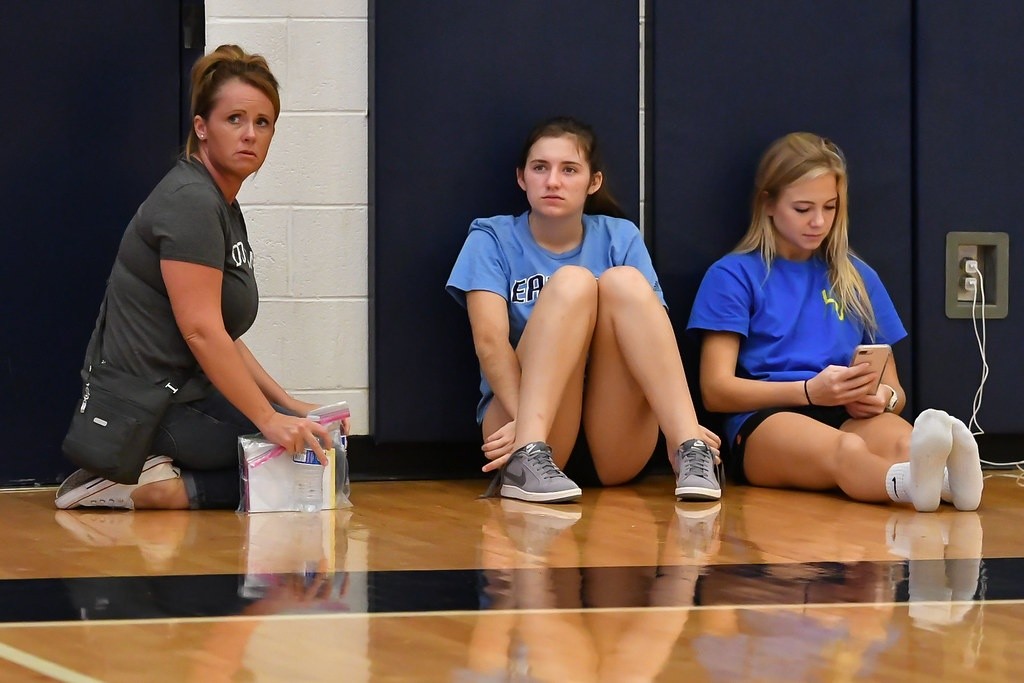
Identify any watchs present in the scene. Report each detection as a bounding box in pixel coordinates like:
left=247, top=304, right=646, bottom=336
left=884, top=384, right=899, bottom=412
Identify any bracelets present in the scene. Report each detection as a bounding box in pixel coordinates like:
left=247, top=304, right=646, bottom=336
left=804, top=379, right=813, bottom=406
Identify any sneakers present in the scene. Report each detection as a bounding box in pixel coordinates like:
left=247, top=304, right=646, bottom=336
left=500, top=498, right=582, bottom=547
left=673, top=439, right=725, bottom=500
left=54, top=452, right=181, bottom=509
left=674, top=501, right=721, bottom=559
left=475, top=442, right=583, bottom=503
left=55, top=510, right=178, bottom=559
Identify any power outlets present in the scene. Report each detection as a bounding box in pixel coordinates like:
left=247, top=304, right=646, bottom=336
left=957, top=246, right=985, bottom=301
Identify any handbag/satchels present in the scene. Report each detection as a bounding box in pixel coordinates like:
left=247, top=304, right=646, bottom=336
left=62, top=364, right=178, bottom=484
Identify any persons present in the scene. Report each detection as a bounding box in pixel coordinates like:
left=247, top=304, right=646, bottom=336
left=446, top=118, right=721, bottom=504
left=54, top=45, right=351, bottom=510
left=685, top=131, right=984, bottom=513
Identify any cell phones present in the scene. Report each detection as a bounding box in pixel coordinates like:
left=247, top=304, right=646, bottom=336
left=848, top=344, right=891, bottom=397
